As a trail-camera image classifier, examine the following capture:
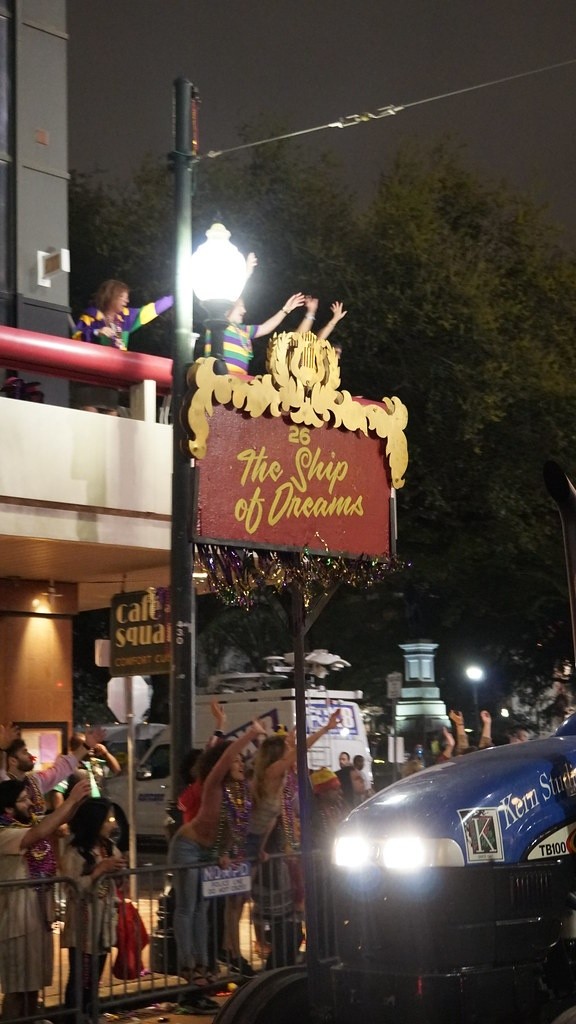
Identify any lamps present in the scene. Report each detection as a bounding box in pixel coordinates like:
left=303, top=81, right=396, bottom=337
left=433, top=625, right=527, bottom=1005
left=42, top=579, right=63, bottom=605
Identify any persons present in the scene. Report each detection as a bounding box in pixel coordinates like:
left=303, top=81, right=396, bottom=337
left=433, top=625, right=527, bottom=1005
left=0, top=724, right=129, bottom=1024
left=399, top=708, right=528, bottom=779
left=70, top=280, right=172, bottom=418
left=296, top=295, right=348, bottom=340
left=169, top=698, right=369, bottom=1015
left=204, top=292, right=305, bottom=374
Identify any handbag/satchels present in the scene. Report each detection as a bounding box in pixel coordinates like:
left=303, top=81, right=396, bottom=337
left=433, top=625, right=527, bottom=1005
left=112, top=900, right=149, bottom=980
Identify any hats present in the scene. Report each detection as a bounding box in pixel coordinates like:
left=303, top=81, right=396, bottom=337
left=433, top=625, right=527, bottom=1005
left=309, top=765, right=341, bottom=796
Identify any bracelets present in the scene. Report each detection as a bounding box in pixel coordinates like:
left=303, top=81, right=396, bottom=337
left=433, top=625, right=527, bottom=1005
left=281, top=306, right=290, bottom=316
left=304, top=314, right=317, bottom=321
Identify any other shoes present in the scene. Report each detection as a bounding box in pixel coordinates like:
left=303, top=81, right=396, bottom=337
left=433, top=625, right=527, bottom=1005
left=178, top=995, right=221, bottom=1015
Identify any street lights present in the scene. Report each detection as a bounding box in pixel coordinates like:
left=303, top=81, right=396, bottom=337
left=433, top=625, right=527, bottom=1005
left=148, top=224, right=253, bottom=974
left=466, top=665, right=483, bottom=734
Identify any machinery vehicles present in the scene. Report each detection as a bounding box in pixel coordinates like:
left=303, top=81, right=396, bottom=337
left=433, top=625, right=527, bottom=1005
left=209, top=712, right=576, bottom=1023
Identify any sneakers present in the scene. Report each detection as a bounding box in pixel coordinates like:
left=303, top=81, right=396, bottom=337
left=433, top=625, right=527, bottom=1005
left=219, top=951, right=259, bottom=980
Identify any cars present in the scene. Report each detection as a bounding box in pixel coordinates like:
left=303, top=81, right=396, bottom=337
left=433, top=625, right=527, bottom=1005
left=76, top=724, right=164, bottom=778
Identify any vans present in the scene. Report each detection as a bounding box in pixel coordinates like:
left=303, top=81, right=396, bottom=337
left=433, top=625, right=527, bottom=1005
left=74, top=653, right=376, bottom=852
